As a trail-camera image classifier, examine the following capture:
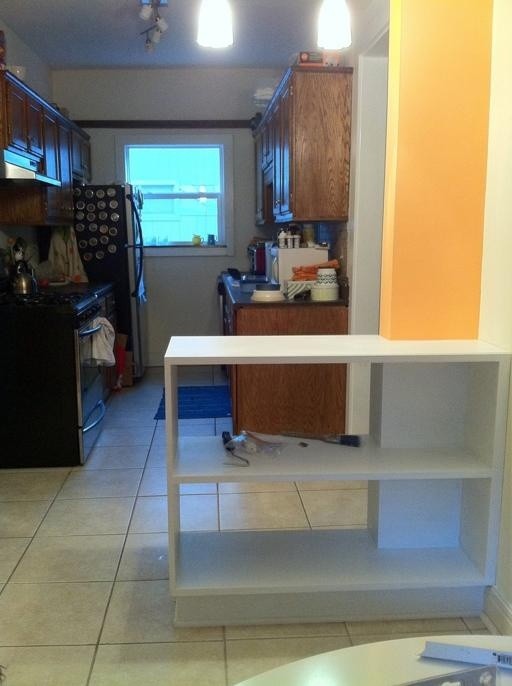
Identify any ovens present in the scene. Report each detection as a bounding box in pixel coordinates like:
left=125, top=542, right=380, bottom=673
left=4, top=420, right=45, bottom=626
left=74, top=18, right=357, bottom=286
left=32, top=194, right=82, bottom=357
left=0, top=316, right=106, bottom=466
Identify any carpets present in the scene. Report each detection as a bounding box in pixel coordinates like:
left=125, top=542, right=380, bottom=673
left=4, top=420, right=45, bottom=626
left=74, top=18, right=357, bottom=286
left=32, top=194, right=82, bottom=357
left=153, top=386, right=234, bottom=418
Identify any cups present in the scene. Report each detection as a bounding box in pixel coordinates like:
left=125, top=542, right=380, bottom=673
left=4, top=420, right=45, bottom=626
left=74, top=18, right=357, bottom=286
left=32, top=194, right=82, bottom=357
left=193, top=235, right=204, bottom=248
left=315, top=268, right=339, bottom=288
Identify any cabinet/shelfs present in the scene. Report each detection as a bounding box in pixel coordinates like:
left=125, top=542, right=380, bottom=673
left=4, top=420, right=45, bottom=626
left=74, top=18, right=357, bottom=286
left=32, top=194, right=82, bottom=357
left=2, top=70, right=91, bottom=227
left=163, top=333, right=508, bottom=624
left=253, top=65, right=353, bottom=225
left=221, top=289, right=347, bottom=437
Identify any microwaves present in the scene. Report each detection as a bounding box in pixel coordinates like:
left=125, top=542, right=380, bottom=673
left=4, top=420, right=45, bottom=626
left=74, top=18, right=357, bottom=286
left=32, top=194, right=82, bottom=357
left=269, top=248, right=328, bottom=289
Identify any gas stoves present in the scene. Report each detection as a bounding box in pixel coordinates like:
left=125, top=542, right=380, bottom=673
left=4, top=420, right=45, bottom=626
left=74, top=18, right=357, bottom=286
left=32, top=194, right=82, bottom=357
left=0, top=290, right=102, bottom=315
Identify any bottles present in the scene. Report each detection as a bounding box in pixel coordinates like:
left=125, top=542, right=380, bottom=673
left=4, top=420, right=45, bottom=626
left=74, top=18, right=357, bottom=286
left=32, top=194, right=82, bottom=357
left=208, top=235, right=215, bottom=248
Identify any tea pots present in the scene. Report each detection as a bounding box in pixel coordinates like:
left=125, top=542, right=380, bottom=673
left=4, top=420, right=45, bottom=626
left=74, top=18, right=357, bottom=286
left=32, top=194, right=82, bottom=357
left=7, top=262, right=36, bottom=298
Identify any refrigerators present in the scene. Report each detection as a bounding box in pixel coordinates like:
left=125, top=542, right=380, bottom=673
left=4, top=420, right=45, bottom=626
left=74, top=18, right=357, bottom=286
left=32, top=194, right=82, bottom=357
left=75, top=185, right=146, bottom=383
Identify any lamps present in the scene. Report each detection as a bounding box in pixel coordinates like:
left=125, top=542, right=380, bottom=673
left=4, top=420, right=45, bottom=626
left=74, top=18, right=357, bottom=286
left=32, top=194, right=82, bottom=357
left=138, top=1, right=170, bottom=55
